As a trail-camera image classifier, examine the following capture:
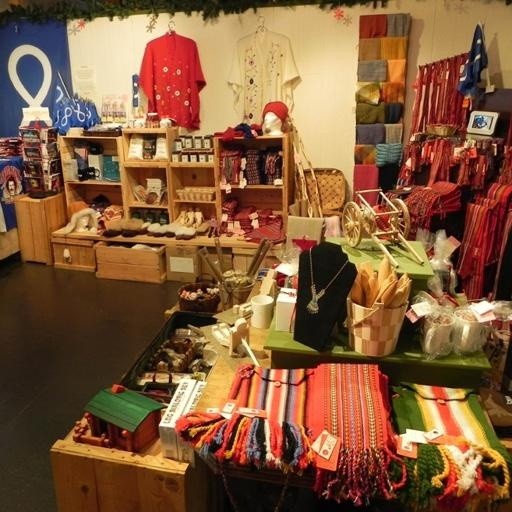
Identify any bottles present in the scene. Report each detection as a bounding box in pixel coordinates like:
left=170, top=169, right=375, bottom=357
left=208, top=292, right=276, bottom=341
left=145, top=112, right=160, bottom=128
left=171, top=134, right=214, bottom=163
left=101, top=104, right=127, bottom=124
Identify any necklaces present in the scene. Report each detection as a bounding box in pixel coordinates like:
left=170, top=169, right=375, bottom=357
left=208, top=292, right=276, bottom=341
left=306, top=245, right=349, bottom=315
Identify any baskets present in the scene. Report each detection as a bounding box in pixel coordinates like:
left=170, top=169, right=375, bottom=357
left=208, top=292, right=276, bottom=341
left=294, top=168, right=346, bottom=210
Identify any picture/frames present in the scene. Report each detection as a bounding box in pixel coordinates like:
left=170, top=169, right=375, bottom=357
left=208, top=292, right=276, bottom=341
left=467, top=111, right=500, bottom=136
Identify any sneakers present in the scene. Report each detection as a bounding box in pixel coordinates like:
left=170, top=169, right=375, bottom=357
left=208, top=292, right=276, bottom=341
left=103, top=218, right=195, bottom=239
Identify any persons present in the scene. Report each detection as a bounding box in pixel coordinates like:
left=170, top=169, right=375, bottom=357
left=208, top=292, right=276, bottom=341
left=72, top=215, right=90, bottom=232
left=262, top=102, right=288, bottom=137
left=6, top=176, right=18, bottom=197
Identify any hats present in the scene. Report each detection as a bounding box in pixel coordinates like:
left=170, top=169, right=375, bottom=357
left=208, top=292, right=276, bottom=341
left=263, top=102, right=288, bottom=122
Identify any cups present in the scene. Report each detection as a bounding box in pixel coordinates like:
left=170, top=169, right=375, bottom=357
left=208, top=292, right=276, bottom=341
left=251, top=294, right=274, bottom=331
left=422, top=313, right=457, bottom=360
left=458, top=310, right=491, bottom=354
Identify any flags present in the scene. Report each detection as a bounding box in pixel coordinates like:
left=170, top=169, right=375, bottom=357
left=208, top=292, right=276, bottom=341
left=456, top=19, right=489, bottom=99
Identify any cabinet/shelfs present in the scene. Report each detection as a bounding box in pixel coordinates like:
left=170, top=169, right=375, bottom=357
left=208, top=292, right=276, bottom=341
left=50, top=377, right=209, bottom=512
